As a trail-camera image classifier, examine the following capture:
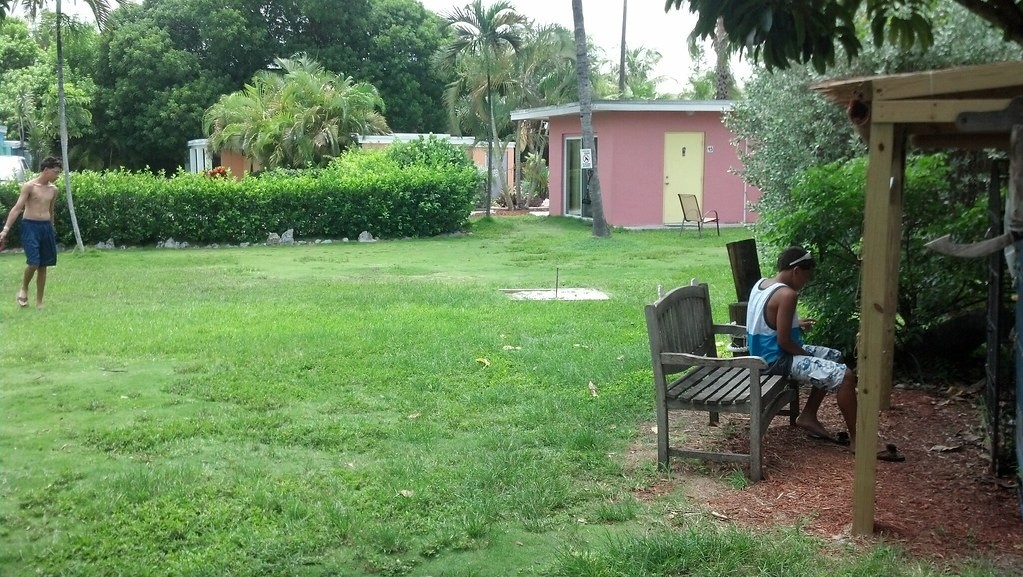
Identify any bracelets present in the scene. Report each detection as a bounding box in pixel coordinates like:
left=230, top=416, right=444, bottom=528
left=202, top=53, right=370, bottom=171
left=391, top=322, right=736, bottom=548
left=3, top=227, right=9, bottom=233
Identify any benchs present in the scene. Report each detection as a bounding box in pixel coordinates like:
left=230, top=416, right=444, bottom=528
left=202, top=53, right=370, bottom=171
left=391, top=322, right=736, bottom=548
left=645, top=278, right=800, bottom=481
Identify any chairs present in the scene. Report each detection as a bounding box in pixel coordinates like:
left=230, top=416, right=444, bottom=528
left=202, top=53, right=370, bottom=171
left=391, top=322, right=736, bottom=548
left=726, top=238, right=761, bottom=355
left=677, top=194, right=720, bottom=238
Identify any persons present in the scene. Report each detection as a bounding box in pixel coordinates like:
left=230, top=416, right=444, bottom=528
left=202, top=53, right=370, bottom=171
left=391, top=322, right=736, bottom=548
left=0, top=155, right=63, bottom=309
left=746, top=245, right=895, bottom=458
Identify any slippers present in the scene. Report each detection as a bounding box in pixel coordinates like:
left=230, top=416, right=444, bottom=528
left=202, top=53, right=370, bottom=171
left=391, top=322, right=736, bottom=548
left=17, top=293, right=29, bottom=307
left=807, top=431, right=850, bottom=446
left=876, top=443, right=906, bottom=463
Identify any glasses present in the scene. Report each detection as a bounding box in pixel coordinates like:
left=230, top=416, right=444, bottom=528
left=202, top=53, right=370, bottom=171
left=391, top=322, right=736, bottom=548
left=789, top=252, right=812, bottom=266
